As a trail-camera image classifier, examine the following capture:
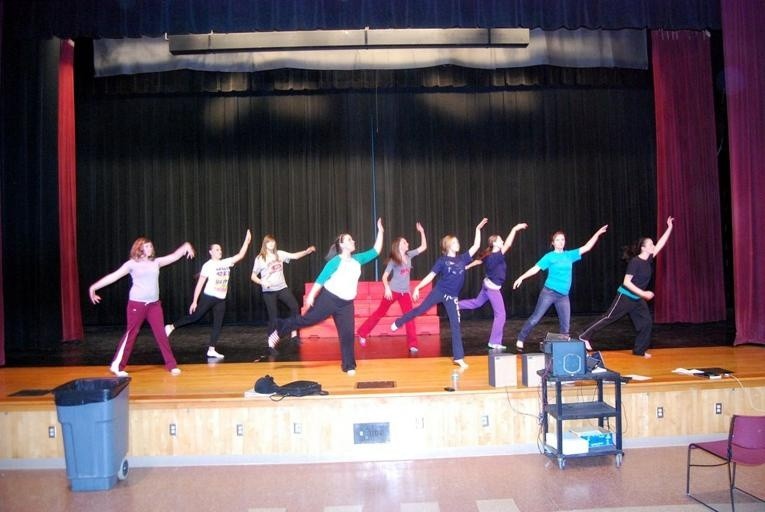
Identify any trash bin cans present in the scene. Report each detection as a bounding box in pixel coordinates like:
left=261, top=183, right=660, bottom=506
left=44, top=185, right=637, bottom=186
left=50, top=377, right=131, bottom=492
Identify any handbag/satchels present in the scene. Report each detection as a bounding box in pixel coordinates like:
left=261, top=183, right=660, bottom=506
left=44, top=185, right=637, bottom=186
left=276, top=381, right=321, bottom=397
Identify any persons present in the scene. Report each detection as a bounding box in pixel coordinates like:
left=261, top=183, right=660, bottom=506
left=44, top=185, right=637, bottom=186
left=88, top=235, right=195, bottom=377
left=578, top=216, right=675, bottom=356
left=457, top=222, right=529, bottom=349
left=391, top=217, right=488, bottom=367
left=165, top=230, right=252, bottom=358
left=268, top=218, right=385, bottom=376
left=251, top=235, right=316, bottom=347
left=514, top=224, right=609, bottom=350
left=358, top=222, right=426, bottom=355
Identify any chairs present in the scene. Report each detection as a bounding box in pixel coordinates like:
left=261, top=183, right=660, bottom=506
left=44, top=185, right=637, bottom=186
left=685, top=413, right=765, bottom=511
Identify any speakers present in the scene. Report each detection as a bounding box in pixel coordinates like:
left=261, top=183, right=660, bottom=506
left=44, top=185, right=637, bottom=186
left=541, top=339, right=587, bottom=376
left=524, top=353, right=576, bottom=386
left=488, top=352, right=517, bottom=388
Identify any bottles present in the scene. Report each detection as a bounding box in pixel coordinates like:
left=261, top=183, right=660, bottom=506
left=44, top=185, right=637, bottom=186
left=451, top=368, right=459, bottom=391
